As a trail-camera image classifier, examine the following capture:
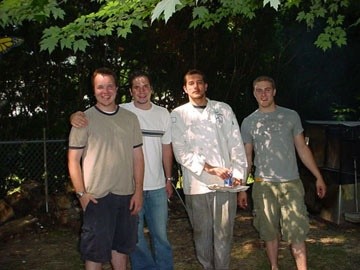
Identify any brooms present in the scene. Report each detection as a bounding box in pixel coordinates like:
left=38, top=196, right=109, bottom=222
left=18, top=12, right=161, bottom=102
left=345, top=159, right=360, bottom=224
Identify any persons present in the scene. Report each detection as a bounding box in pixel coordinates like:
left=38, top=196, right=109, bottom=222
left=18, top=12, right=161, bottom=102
left=69, top=71, right=174, bottom=270
left=237, top=76, right=327, bottom=270
left=169, top=70, right=249, bottom=270
left=67, top=69, right=145, bottom=270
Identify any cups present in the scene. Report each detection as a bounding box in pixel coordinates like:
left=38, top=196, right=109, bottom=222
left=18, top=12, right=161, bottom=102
left=224, top=171, right=232, bottom=187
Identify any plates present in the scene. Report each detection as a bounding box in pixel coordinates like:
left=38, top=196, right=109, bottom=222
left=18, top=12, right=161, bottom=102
left=208, top=184, right=250, bottom=193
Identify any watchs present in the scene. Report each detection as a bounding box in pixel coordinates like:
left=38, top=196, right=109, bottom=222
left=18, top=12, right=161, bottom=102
left=75, top=191, right=86, bottom=198
left=166, top=176, right=175, bottom=181
